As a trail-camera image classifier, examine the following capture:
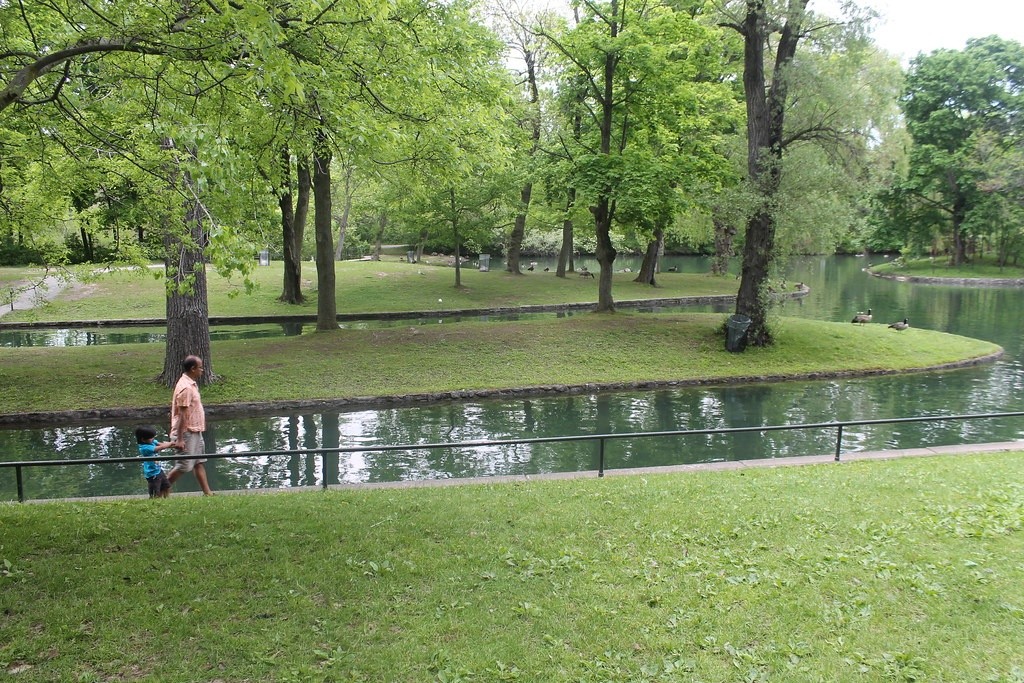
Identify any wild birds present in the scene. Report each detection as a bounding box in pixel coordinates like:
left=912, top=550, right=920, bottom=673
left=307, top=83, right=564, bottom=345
left=855, top=252, right=934, bottom=268
left=735, top=271, right=910, bottom=334
left=400, top=252, right=595, bottom=279
left=618, top=265, right=678, bottom=273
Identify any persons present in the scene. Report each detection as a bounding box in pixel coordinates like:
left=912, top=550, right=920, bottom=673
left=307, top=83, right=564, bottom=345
left=135, top=425, right=175, bottom=498
left=160, top=355, right=217, bottom=497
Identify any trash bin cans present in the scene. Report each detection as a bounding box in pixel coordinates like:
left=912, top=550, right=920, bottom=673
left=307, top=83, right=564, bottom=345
left=479, top=253, right=490, bottom=272
left=258, top=250, right=270, bottom=265
left=406, top=251, right=414, bottom=263
